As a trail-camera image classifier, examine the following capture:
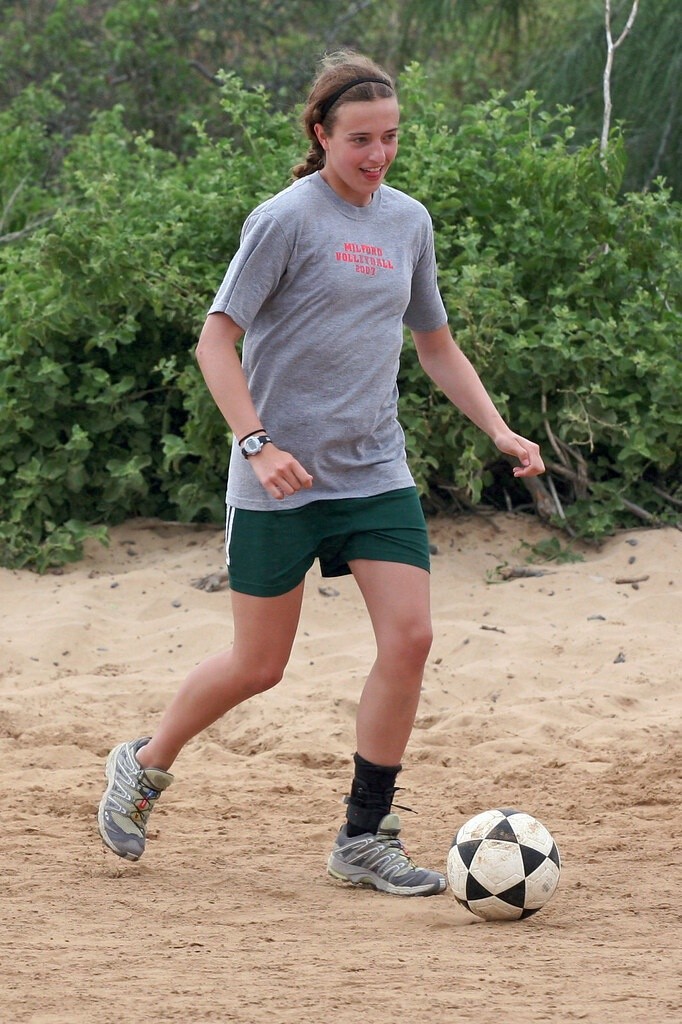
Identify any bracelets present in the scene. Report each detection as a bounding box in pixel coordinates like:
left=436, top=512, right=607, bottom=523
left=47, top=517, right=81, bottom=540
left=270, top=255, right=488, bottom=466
left=239, top=429, right=266, bottom=446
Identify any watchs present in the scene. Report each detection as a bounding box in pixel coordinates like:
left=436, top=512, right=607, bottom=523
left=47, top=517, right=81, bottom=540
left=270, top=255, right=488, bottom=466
left=242, top=436, right=273, bottom=460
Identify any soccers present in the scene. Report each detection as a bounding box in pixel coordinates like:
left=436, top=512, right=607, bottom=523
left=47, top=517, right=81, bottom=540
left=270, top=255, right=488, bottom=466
left=446, top=807, right=561, bottom=922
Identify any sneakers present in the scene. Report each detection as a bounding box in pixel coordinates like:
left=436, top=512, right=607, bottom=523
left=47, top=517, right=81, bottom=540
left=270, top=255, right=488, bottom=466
left=327, top=813, right=445, bottom=895
left=98, top=737, right=173, bottom=862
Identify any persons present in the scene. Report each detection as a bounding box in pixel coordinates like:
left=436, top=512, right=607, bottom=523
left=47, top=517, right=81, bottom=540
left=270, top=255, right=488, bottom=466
left=97, top=53, right=548, bottom=899
